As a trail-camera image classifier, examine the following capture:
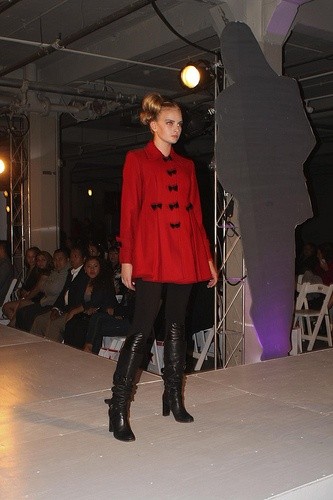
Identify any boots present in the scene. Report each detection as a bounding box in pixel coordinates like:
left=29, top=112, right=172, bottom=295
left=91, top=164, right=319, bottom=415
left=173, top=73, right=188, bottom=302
left=104, top=331, right=155, bottom=441
left=162, top=323, right=196, bottom=422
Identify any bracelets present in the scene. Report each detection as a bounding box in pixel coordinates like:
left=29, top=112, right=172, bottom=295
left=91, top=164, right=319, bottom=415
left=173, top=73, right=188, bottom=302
left=23, top=298, right=24, bottom=300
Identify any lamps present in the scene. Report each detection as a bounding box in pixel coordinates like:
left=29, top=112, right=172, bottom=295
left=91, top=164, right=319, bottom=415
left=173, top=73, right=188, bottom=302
left=177, top=59, right=217, bottom=93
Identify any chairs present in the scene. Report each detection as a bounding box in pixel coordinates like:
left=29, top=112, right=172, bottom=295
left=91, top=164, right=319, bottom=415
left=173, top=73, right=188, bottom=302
left=286, top=282, right=333, bottom=352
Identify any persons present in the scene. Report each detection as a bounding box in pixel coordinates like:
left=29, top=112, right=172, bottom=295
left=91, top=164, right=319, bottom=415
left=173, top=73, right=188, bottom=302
left=63, top=256, right=112, bottom=349
left=294, top=241, right=333, bottom=337
left=0, top=240, right=136, bottom=355
left=103, top=93, right=219, bottom=442
